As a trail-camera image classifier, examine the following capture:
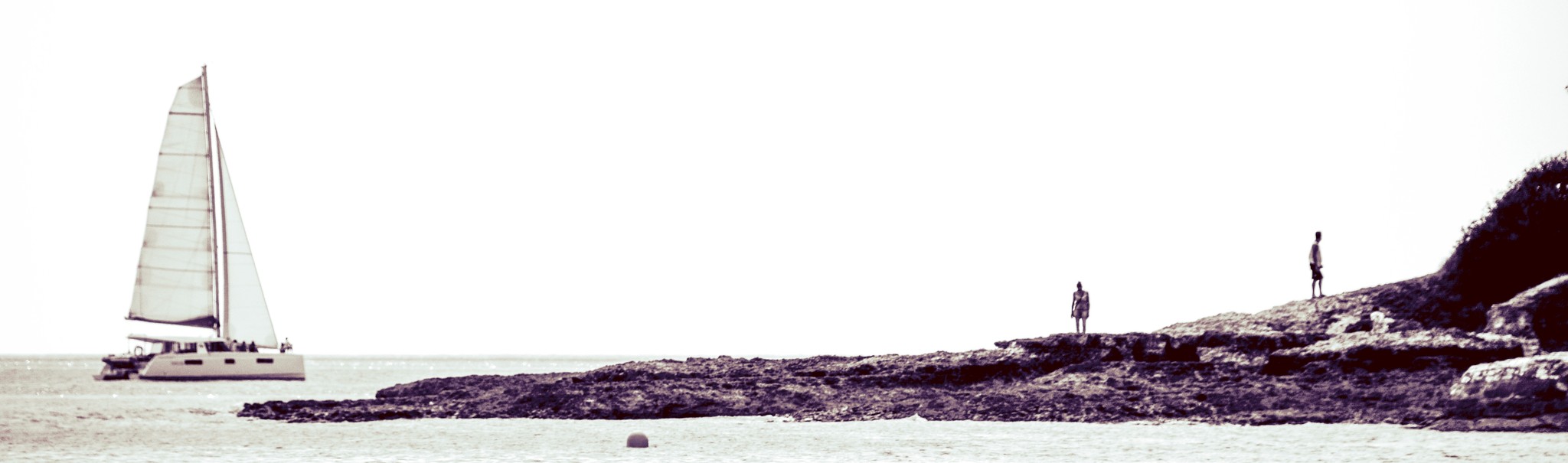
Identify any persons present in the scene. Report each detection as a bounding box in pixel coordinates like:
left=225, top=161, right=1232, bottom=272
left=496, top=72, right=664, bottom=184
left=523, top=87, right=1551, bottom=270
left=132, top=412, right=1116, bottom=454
left=230, top=340, right=259, bottom=352
left=280, top=338, right=290, bottom=352
left=1071, top=282, right=1090, bottom=334
left=1309, top=232, right=1326, bottom=297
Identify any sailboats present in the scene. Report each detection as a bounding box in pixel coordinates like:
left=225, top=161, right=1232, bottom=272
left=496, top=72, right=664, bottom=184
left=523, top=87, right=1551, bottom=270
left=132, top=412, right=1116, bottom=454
left=92, top=61, right=309, bottom=384
